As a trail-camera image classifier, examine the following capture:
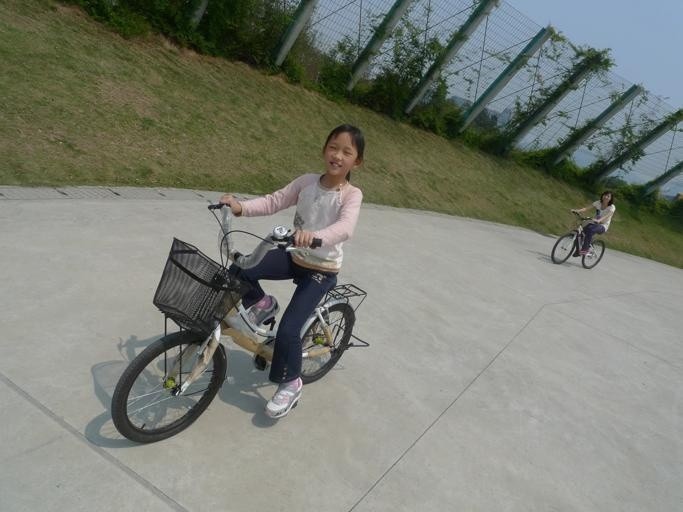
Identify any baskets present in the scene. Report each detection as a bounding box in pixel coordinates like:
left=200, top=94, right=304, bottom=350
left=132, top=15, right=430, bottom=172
left=153, top=237, right=254, bottom=337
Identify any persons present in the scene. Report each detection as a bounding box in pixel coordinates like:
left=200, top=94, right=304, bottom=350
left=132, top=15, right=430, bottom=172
left=570, top=191, right=616, bottom=257
left=219, top=125, right=363, bottom=419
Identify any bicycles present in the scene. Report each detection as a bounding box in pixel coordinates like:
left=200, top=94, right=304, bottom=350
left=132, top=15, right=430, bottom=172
left=110, top=193, right=371, bottom=446
left=547, top=209, right=606, bottom=268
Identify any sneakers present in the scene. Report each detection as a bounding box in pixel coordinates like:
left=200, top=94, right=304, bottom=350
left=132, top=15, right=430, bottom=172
left=248, top=295, right=279, bottom=326
left=264, top=377, right=303, bottom=419
